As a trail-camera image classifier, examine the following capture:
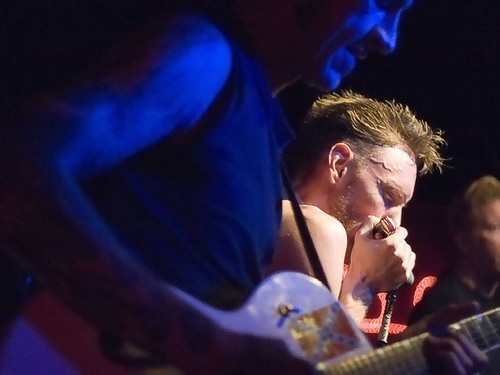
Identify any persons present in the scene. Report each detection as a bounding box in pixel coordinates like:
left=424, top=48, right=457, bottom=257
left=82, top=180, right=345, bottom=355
left=0, top=1, right=417, bottom=375
left=264, top=89, right=443, bottom=333
left=413, top=176, right=500, bottom=324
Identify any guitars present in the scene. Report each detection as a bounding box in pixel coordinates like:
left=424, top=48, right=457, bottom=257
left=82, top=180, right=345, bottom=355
left=0, top=271, right=500, bottom=374
